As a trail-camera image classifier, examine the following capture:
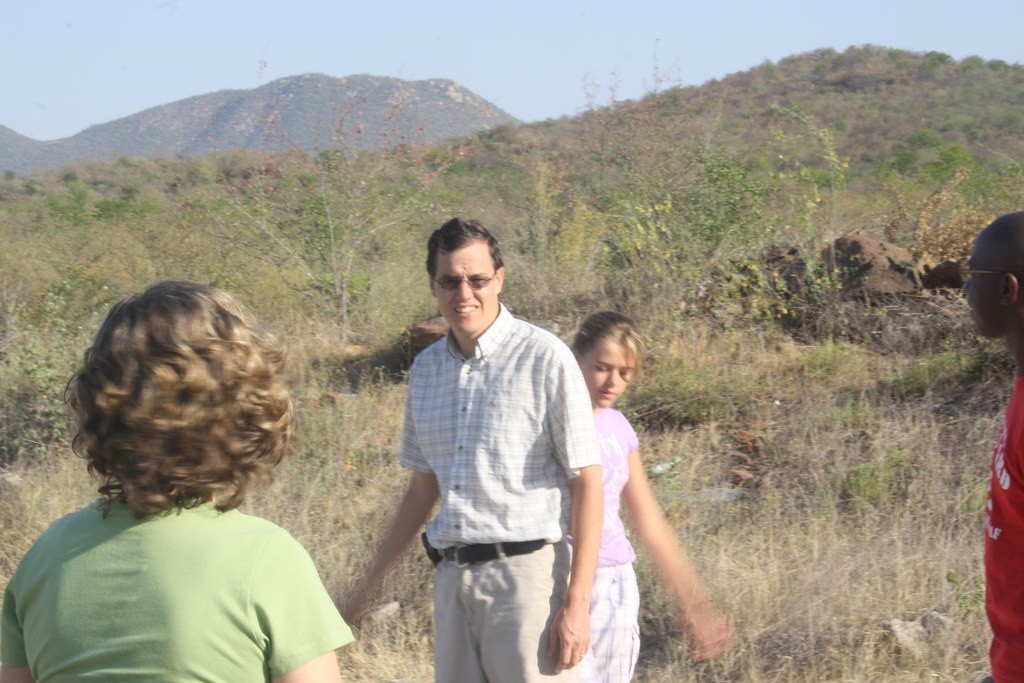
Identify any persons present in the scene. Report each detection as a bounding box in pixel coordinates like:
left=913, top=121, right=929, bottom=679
left=338, top=218, right=604, bottom=683
left=572, top=310, right=722, bottom=683
left=963, top=211, right=1024, bottom=683
left=0, top=279, right=356, bottom=683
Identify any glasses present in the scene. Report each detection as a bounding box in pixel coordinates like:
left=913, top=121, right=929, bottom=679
left=958, top=264, right=1022, bottom=287
left=434, top=267, right=497, bottom=290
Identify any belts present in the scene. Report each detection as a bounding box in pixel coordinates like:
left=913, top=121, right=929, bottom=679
left=438, top=539, right=545, bottom=566
